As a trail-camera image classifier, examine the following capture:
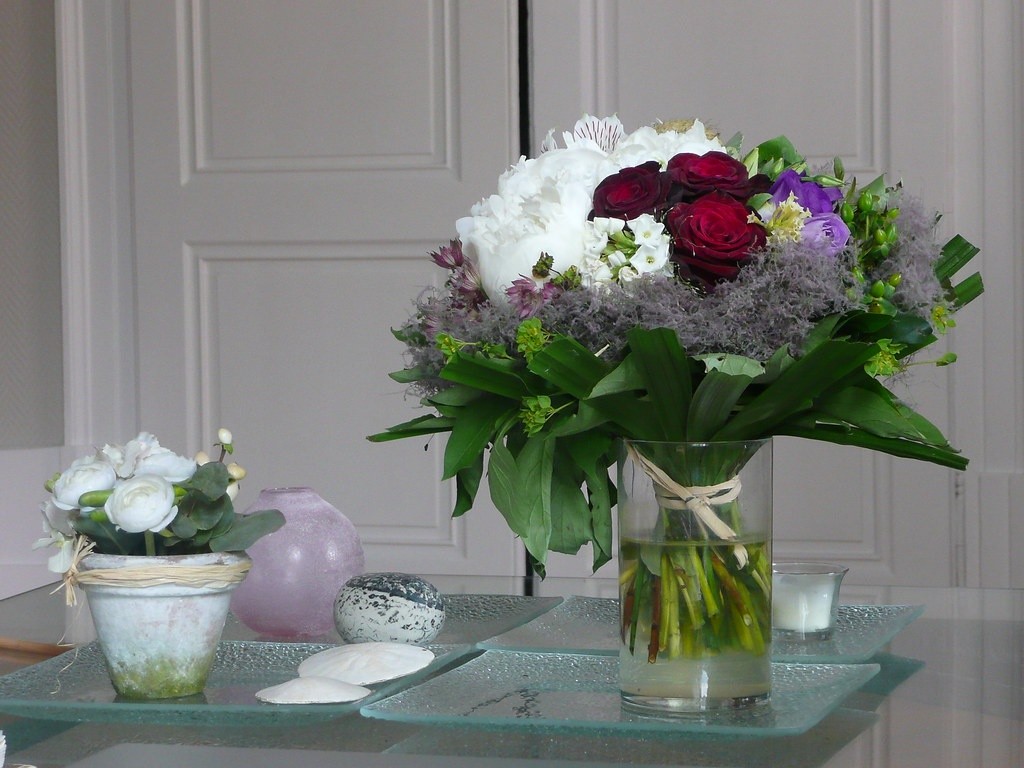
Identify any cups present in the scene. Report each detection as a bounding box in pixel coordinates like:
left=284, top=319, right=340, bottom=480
left=772, top=561, right=850, bottom=640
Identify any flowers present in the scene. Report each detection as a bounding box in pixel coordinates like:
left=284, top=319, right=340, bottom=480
left=363, top=114, right=985, bottom=665
left=31, top=427, right=286, bottom=575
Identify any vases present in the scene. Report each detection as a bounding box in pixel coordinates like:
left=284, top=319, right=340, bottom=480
left=231, top=487, right=364, bottom=637
left=69, top=550, right=255, bottom=701
left=609, top=436, right=777, bottom=721
